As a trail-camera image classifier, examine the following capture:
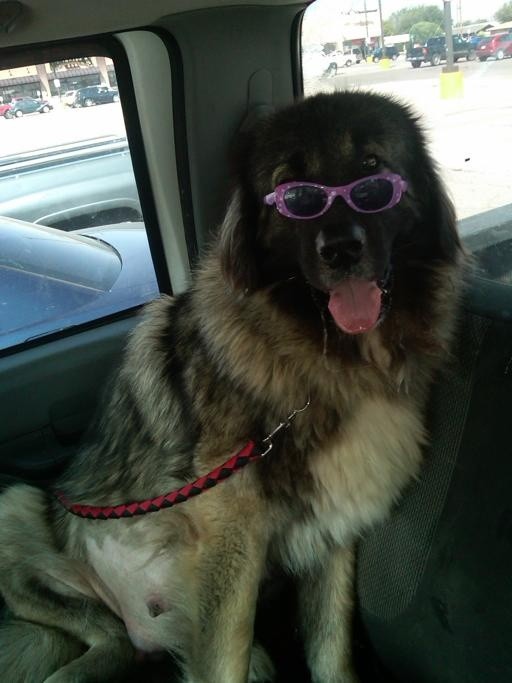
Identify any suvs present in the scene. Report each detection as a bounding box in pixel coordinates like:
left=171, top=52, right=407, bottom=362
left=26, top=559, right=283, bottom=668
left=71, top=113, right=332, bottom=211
left=371, top=47, right=399, bottom=62
left=477, top=34, right=512, bottom=61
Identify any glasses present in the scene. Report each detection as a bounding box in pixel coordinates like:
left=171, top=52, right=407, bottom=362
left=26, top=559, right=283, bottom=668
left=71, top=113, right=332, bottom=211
left=264, top=173, right=408, bottom=219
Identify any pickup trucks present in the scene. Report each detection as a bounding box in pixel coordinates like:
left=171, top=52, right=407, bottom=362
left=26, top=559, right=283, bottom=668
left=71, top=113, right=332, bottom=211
left=0, top=97, right=25, bottom=119
left=406, top=35, right=476, bottom=68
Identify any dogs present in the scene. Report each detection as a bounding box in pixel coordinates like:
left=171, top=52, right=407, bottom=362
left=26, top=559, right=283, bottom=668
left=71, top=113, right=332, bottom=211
left=0, top=89, right=486, bottom=683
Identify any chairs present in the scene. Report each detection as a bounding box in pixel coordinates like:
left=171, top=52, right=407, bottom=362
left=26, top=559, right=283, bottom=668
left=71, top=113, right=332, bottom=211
left=8, top=272, right=511, bottom=683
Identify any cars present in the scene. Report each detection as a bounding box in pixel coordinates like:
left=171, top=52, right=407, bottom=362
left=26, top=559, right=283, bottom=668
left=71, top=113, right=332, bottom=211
left=64, top=87, right=119, bottom=108
left=328, top=47, right=362, bottom=68
left=8, top=100, right=53, bottom=118
